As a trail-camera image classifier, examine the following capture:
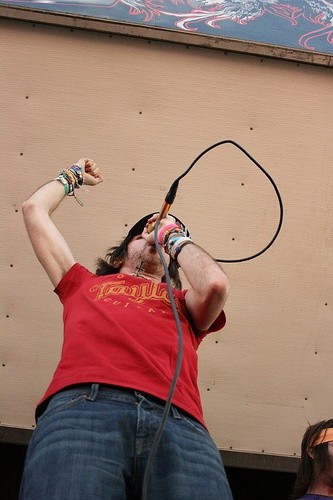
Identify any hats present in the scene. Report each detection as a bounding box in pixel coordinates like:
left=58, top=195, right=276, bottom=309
left=126, top=211, right=189, bottom=239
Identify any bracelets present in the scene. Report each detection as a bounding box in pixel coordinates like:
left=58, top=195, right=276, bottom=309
left=157, top=223, right=199, bottom=261
left=55, top=163, right=89, bottom=206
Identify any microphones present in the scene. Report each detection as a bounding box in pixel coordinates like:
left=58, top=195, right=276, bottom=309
left=147, top=178, right=179, bottom=234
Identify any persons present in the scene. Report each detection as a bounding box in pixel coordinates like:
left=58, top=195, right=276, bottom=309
left=288, top=419, right=333, bottom=500
left=20, top=157, right=231, bottom=500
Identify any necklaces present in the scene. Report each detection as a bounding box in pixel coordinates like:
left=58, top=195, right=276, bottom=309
left=130, top=261, right=153, bottom=280
